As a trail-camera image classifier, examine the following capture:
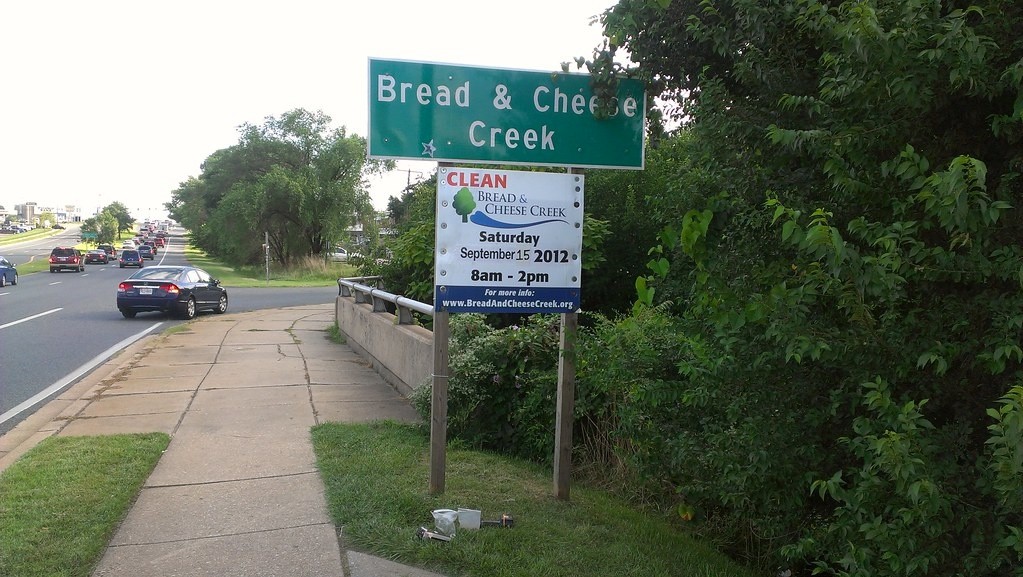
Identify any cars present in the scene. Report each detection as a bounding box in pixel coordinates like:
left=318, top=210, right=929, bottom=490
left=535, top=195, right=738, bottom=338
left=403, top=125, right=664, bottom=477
left=144, top=240, right=158, bottom=256
left=137, top=245, right=155, bottom=261
left=0, top=223, right=37, bottom=235
left=85, top=249, right=109, bottom=265
left=328, top=245, right=364, bottom=262
left=131, top=219, right=173, bottom=249
left=122, top=240, right=135, bottom=249
left=0, top=255, right=18, bottom=287
left=52, top=224, right=65, bottom=231
left=97, top=244, right=118, bottom=261
left=115, top=266, right=228, bottom=319
left=119, top=249, right=144, bottom=269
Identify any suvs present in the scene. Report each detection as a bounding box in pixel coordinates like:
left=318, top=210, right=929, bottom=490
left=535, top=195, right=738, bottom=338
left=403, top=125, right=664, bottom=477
left=48, top=246, right=85, bottom=272
